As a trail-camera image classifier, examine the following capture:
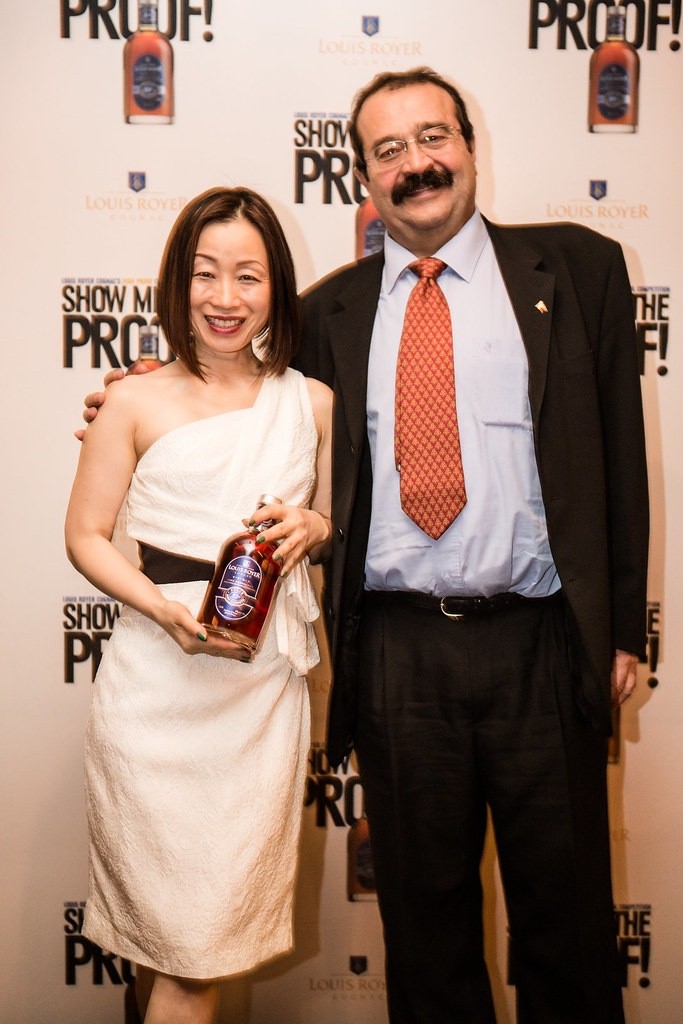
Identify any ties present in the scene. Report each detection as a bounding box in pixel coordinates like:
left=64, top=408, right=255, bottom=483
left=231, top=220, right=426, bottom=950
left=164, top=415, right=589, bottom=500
left=393, top=256, right=468, bottom=541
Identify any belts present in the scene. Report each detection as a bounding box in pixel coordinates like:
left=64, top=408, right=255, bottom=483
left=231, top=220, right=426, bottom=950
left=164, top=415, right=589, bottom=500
left=359, top=591, right=566, bottom=616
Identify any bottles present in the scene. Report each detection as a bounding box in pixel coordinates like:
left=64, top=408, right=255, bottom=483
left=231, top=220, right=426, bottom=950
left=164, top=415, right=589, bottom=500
left=197, top=494, right=285, bottom=656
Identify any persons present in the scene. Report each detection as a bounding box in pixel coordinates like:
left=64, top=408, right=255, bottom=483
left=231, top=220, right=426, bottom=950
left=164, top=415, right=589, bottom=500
left=74, top=67, right=652, bottom=1024
left=65, top=184, right=332, bottom=1024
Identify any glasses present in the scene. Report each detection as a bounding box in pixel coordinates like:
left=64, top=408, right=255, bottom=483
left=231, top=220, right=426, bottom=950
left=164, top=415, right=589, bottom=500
left=363, top=125, right=463, bottom=171
